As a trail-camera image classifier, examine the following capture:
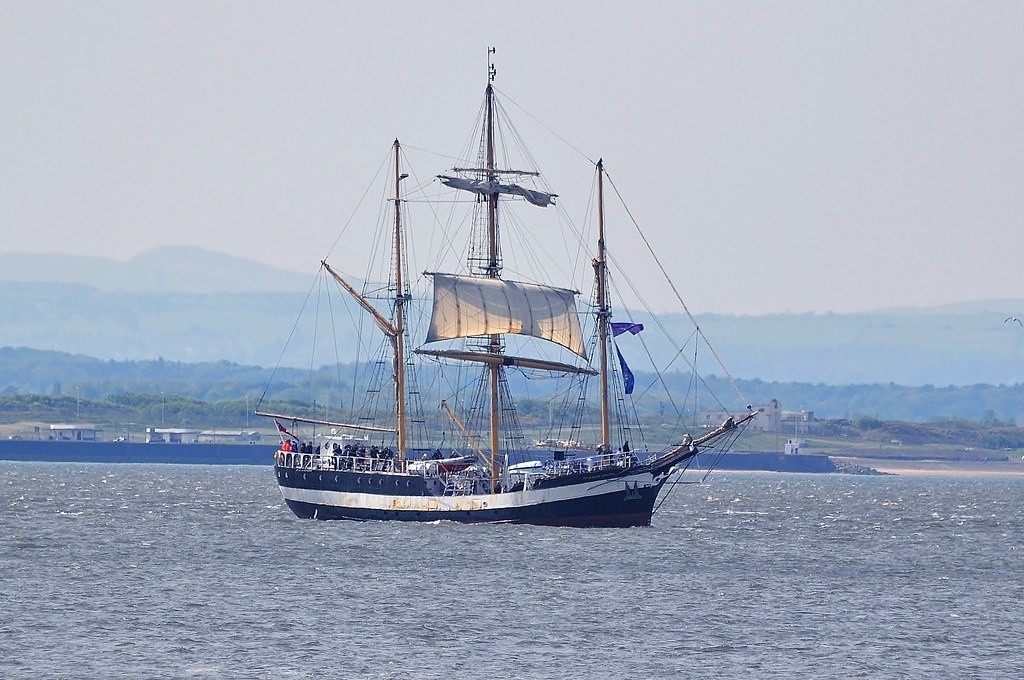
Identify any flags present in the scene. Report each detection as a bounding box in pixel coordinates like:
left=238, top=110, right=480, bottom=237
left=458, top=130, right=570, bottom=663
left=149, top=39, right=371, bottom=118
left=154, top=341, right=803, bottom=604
left=276, top=420, right=286, bottom=433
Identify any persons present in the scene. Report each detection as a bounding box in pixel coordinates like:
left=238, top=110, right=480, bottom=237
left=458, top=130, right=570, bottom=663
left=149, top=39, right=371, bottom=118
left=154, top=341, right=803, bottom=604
left=434, top=450, right=442, bottom=459
left=450, top=451, right=459, bottom=458
left=291, top=441, right=297, bottom=465
left=616, top=448, right=621, bottom=458
left=622, top=441, right=632, bottom=464
left=596, top=445, right=603, bottom=455
left=306, top=442, right=313, bottom=462
left=283, top=440, right=291, bottom=462
left=421, top=453, right=430, bottom=460
left=332, top=443, right=394, bottom=471
left=605, top=447, right=613, bottom=458
left=300, top=443, right=307, bottom=466
left=316, top=442, right=322, bottom=468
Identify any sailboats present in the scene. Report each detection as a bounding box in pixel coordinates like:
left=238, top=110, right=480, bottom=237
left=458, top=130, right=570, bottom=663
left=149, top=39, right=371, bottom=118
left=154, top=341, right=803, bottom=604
left=253, top=45, right=764, bottom=528
